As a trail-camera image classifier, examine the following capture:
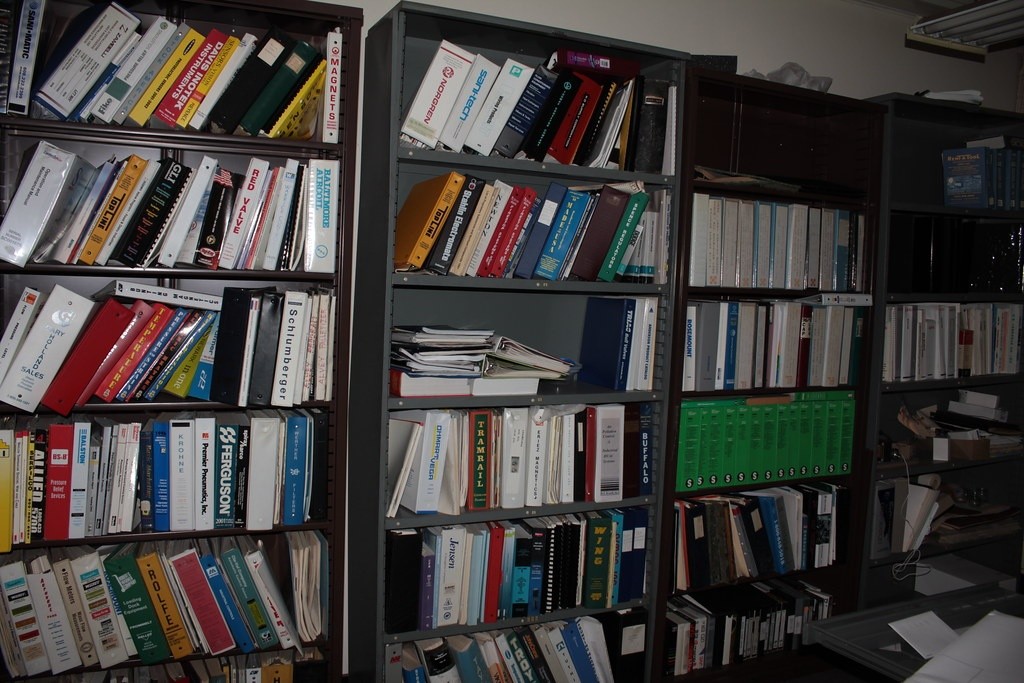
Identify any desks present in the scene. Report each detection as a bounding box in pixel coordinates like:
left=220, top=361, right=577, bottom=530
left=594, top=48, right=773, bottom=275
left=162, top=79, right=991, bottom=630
left=804, top=577, right=1023, bottom=682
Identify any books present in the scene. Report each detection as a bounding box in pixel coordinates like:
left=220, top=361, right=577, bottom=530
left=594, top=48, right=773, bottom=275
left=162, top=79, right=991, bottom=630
left=0, top=140, right=339, bottom=274
left=385, top=507, right=647, bottom=683
left=930, top=389, right=1024, bottom=462
left=400, top=39, right=677, bottom=176
left=394, top=172, right=672, bottom=284
left=941, top=135, right=1024, bottom=216
left=882, top=302, right=1024, bottom=383
left=0, top=280, right=337, bottom=407
left=665, top=482, right=849, bottom=677
left=386, top=403, right=652, bottom=518
left=682, top=293, right=872, bottom=392
left=0, top=0, right=342, bottom=145
left=675, top=390, right=856, bottom=493
left=0, top=408, right=329, bottom=553
left=688, top=192, right=878, bottom=295
left=869, top=476, right=941, bottom=560
left=0, top=530, right=329, bottom=683
left=389, top=294, right=657, bottom=397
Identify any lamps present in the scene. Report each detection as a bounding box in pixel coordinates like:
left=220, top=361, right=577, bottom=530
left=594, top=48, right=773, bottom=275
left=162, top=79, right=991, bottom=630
left=905, top=0, right=1024, bottom=56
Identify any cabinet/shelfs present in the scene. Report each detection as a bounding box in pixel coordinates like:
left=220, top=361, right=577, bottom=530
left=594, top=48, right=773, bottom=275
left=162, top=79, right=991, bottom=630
left=0, top=0, right=363, bottom=682
left=852, top=90, right=1023, bottom=614
left=654, top=60, right=886, bottom=680
left=349, top=0, right=693, bottom=682
